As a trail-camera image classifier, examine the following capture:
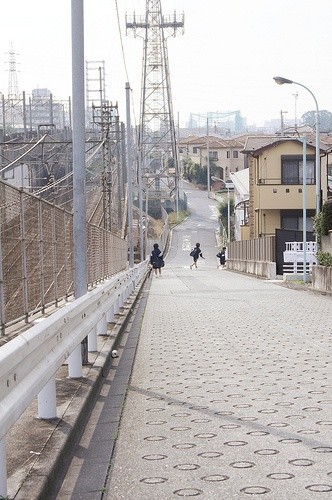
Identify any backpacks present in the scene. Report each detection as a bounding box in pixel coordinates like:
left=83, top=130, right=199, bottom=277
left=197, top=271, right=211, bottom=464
left=217, top=251, right=223, bottom=258
left=190, top=249, right=199, bottom=257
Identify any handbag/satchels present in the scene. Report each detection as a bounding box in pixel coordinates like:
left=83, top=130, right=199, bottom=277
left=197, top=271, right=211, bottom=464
left=159, top=261, right=164, bottom=267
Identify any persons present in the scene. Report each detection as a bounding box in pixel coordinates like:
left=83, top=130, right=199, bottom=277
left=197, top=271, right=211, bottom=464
left=217, top=247, right=226, bottom=269
left=189, top=243, right=205, bottom=270
left=151, top=243, right=163, bottom=278
